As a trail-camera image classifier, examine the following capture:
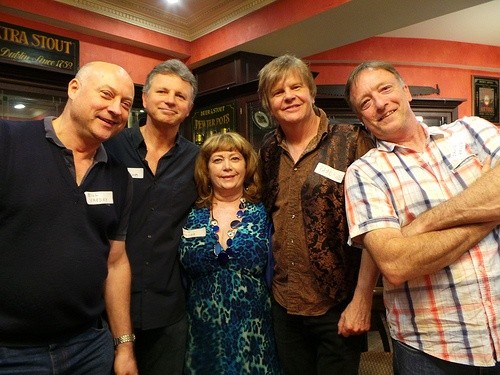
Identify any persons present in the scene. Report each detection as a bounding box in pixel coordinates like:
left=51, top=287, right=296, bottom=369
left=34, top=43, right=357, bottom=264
left=0, top=61, right=139, bottom=375
left=102, top=59, right=201, bottom=375
left=259, top=55, right=379, bottom=375
left=344, top=62, right=500, bottom=375
left=178, top=132, right=280, bottom=375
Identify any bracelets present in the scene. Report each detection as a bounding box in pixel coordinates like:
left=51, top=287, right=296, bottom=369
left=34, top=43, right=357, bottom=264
left=113, top=334, right=136, bottom=344
